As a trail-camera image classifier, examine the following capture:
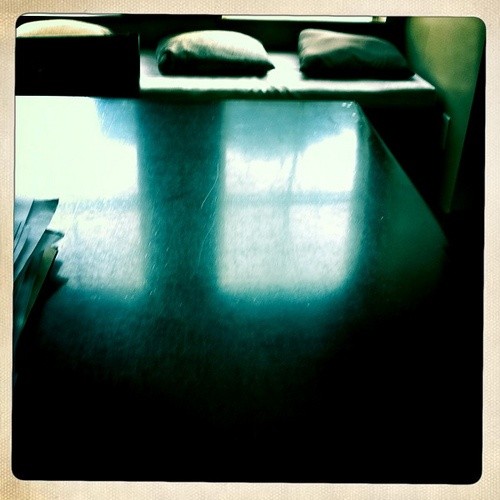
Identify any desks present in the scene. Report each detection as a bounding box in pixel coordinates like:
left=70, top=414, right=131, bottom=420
left=15, top=92, right=478, bottom=486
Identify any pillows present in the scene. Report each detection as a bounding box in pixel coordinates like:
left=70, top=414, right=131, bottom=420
left=154, top=30, right=276, bottom=79
left=297, top=27, right=415, bottom=81
